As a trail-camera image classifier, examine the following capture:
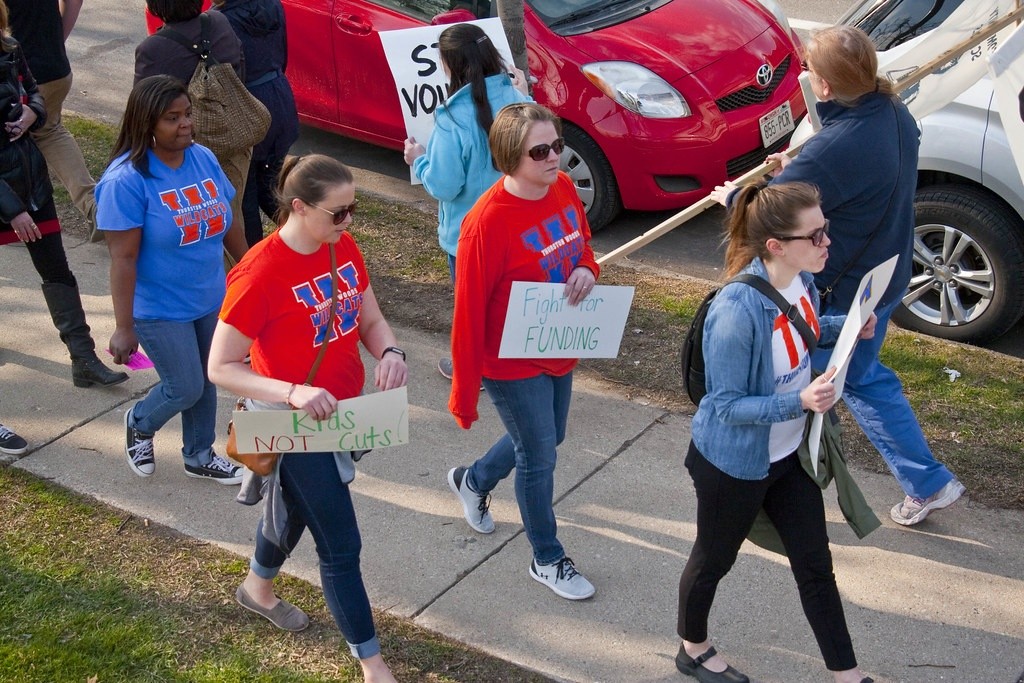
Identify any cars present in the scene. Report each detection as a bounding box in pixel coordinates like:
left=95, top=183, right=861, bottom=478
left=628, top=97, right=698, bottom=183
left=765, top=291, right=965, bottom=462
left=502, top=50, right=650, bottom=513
left=144, top=1, right=813, bottom=233
left=786, top=0, right=1023, bottom=345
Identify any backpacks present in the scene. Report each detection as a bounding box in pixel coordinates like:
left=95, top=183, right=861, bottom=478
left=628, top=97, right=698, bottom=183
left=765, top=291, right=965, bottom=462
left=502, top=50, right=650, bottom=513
left=153, top=12, right=272, bottom=163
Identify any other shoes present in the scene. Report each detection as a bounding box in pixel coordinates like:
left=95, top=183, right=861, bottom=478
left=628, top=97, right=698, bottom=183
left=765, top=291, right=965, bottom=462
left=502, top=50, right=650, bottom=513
left=236, top=582, right=309, bottom=631
left=676, top=641, right=750, bottom=683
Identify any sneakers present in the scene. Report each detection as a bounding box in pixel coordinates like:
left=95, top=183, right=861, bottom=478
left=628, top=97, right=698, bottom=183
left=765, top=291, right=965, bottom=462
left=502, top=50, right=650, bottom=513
left=447, top=466, right=495, bottom=533
left=528, top=550, right=595, bottom=600
left=86, top=202, right=105, bottom=243
left=439, top=357, right=453, bottom=378
left=890, top=476, right=967, bottom=525
left=124, top=407, right=155, bottom=477
left=183, top=451, right=243, bottom=485
left=0, top=424, right=28, bottom=454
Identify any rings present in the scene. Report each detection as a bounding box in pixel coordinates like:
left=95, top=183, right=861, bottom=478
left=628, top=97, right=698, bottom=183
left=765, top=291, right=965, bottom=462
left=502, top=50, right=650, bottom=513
left=14, top=230, right=19, bottom=233
left=30, top=222, right=37, bottom=230
left=821, top=373, right=828, bottom=382
left=824, top=392, right=831, bottom=397
left=583, top=286, right=588, bottom=289
left=19, top=118, right=22, bottom=122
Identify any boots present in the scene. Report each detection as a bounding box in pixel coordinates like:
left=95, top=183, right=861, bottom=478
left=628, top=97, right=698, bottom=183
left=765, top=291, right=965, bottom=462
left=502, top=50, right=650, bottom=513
left=41, top=276, right=129, bottom=388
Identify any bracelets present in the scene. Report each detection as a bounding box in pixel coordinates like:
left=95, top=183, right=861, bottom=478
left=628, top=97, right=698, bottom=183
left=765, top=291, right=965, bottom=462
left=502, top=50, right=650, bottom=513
left=381, top=347, right=407, bottom=360
left=286, top=382, right=296, bottom=404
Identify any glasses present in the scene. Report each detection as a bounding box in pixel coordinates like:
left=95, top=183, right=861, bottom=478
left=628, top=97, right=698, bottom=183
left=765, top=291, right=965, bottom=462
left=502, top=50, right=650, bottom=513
left=765, top=219, right=830, bottom=247
left=516, top=137, right=565, bottom=161
left=290, top=197, right=359, bottom=225
left=800, top=61, right=809, bottom=70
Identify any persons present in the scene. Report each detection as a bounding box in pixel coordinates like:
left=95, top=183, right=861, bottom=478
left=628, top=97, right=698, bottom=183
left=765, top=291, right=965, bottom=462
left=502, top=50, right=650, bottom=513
left=710, top=25, right=968, bottom=527
left=93, top=74, right=250, bottom=485
left=675, top=176, right=877, bottom=683
left=0, top=0, right=129, bottom=456
left=209, top=154, right=406, bottom=683
left=133, top=0, right=300, bottom=272
left=446, top=102, right=600, bottom=600
left=404, top=24, right=533, bottom=390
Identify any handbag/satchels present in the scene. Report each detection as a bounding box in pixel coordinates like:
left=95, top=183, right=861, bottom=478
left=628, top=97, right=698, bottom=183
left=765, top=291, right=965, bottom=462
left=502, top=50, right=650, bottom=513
left=226, top=402, right=280, bottom=476
left=682, top=286, right=721, bottom=407
